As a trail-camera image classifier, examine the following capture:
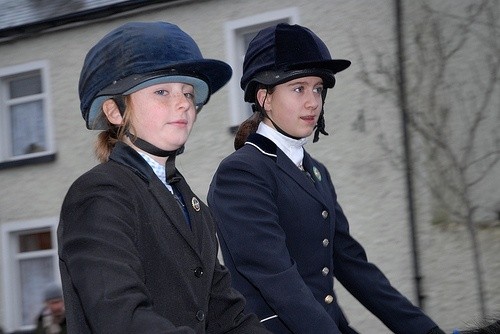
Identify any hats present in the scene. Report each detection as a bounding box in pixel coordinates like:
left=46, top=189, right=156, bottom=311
left=238, top=23, right=351, bottom=101
left=77, top=20, right=234, bottom=130
left=43, top=284, right=62, bottom=300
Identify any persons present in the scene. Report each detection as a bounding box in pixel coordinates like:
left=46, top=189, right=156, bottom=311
left=57, top=21, right=274, bottom=334
left=35, top=287, right=65, bottom=334
left=206, top=24, right=444, bottom=334
